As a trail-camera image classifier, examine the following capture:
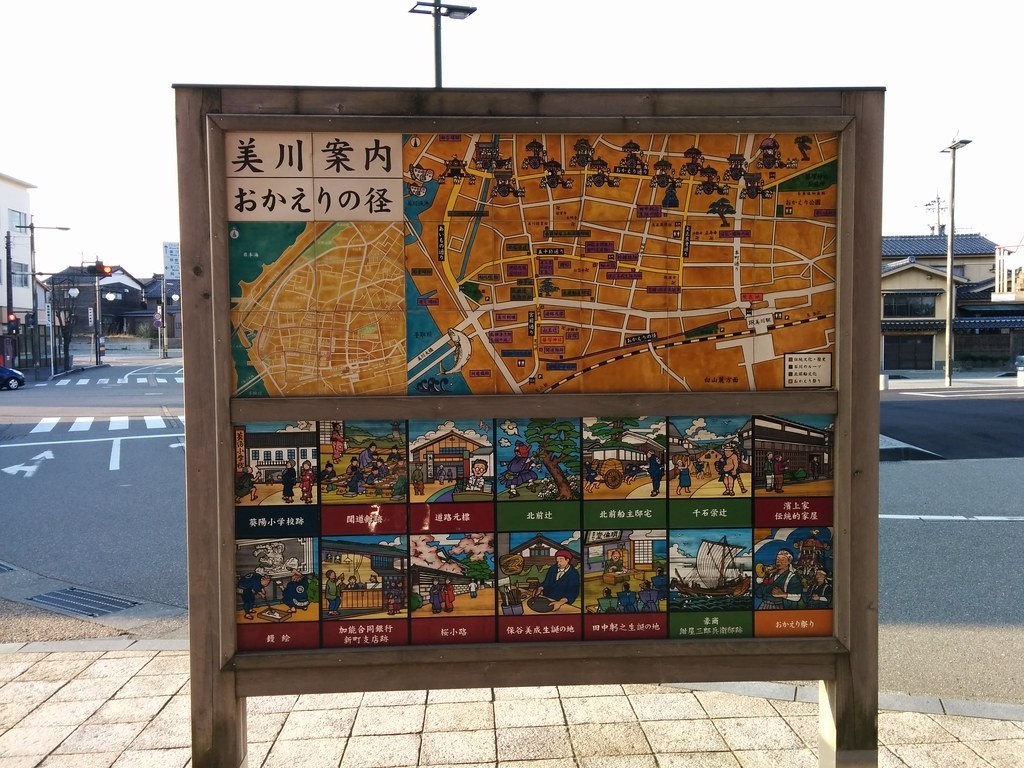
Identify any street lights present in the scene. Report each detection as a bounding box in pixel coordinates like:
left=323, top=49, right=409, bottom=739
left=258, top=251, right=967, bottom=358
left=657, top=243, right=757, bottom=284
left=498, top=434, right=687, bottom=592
left=939, top=139, right=973, bottom=386
left=163, top=277, right=179, bottom=359
left=96, top=277, right=115, bottom=365
left=924, top=194, right=948, bottom=238
left=51, top=276, right=79, bottom=374
left=15, top=224, right=71, bottom=382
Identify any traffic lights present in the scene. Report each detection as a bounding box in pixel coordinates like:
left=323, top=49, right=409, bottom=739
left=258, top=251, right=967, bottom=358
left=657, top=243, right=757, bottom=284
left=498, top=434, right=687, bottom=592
left=87, top=264, right=112, bottom=274
left=8, top=314, right=19, bottom=335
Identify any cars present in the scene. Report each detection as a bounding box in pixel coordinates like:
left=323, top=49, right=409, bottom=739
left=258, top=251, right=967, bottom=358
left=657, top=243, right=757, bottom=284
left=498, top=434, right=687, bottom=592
left=0, top=365, right=25, bottom=390
